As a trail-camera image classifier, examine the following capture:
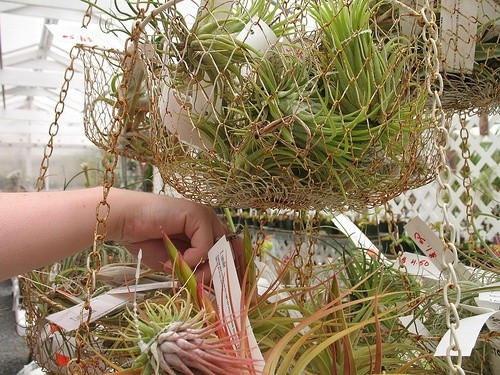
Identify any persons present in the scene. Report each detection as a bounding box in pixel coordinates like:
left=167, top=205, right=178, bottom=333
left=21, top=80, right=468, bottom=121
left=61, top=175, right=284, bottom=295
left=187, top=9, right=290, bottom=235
left=1, top=186, right=245, bottom=294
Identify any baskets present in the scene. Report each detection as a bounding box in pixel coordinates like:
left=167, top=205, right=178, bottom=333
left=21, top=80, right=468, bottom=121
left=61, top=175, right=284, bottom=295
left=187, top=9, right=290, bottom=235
left=17, top=273, right=139, bottom=374
left=118, top=0, right=449, bottom=213
left=77, top=46, right=158, bottom=166
